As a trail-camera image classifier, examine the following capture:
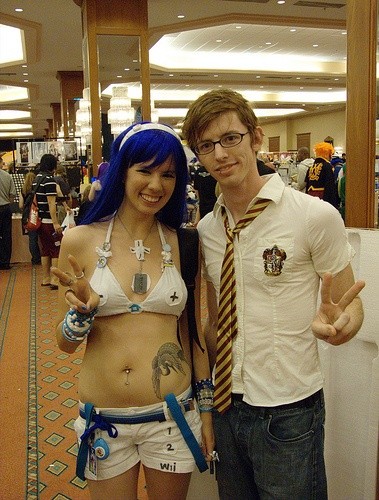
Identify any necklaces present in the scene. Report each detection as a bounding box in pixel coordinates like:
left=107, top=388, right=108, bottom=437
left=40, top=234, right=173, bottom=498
left=116, top=213, right=156, bottom=295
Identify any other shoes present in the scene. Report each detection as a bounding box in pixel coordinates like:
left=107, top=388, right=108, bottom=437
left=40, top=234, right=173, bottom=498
left=50, top=284, right=58, bottom=290
left=41, top=283, right=51, bottom=286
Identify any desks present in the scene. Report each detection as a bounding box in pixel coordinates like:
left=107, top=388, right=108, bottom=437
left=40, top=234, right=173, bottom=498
left=10, top=216, right=32, bottom=264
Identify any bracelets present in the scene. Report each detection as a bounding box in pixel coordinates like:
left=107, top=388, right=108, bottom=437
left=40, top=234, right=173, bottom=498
left=62, top=306, right=99, bottom=343
left=193, top=377, right=216, bottom=413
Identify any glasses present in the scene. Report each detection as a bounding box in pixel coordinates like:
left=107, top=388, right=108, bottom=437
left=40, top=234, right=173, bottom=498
left=192, top=129, right=251, bottom=156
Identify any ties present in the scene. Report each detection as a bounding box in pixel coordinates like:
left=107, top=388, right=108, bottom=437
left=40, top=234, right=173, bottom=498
left=212, top=198, right=271, bottom=415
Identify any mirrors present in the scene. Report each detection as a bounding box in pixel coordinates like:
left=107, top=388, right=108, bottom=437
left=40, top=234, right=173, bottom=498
left=88, top=34, right=151, bottom=169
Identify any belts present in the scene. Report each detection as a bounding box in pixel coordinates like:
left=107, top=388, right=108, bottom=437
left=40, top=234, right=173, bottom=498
left=79, top=392, right=195, bottom=424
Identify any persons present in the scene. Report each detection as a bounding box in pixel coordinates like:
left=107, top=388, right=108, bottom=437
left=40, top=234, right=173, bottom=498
left=0, top=141, right=346, bottom=290
left=181, top=87, right=367, bottom=500
left=50, top=121, right=215, bottom=500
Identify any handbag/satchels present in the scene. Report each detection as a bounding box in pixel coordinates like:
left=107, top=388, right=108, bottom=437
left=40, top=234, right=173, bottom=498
left=21, top=174, right=47, bottom=232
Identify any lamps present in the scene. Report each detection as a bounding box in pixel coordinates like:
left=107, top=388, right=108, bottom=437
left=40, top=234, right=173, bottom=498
left=108, top=86, right=136, bottom=143
left=76, top=88, right=92, bottom=137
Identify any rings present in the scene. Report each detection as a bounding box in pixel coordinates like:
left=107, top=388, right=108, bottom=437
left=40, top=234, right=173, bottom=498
left=73, top=271, right=84, bottom=279
left=64, top=288, right=74, bottom=296
left=59, top=274, right=73, bottom=287
